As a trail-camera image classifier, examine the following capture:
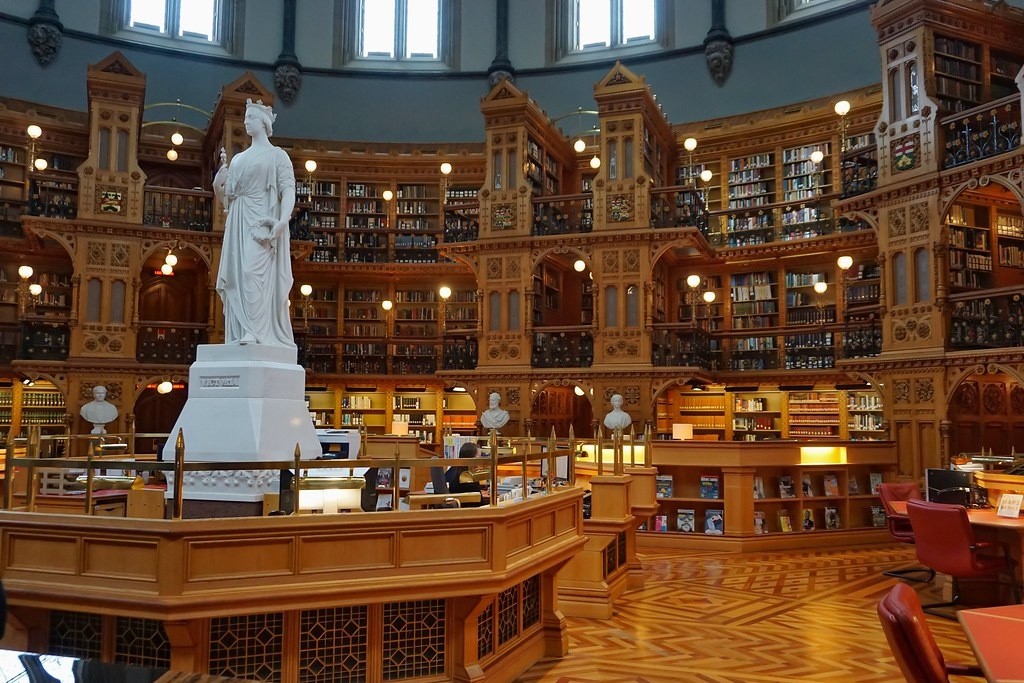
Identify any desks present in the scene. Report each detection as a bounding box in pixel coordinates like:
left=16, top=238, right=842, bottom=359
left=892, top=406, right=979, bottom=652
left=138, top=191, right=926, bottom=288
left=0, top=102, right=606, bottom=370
left=957, top=605, right=1024, bottom=683
left=941, top=508, right=1024, bottom=603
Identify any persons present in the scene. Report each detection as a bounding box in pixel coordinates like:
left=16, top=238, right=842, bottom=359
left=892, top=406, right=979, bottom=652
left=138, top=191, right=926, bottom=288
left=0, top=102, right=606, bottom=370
left=80, top=385, right=119, bottom=424
left=212, top=98, right=295, bottom=346
left=481, top=391, right=510, bottom=428
left=439, top=441, right=489, bottom=507
left=603, top=393, right=632, bottom=429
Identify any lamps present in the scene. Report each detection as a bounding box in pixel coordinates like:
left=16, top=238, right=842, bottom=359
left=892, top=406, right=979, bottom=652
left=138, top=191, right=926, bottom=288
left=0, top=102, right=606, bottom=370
left=382, top=300, right=393, bottom=338
left=810, top=151, right=824, bottom=197
left=300, top=285, right=313, bottom=331
left=167, top=117, right=178, bottom=161
left=590, top=125, right=601, bottom=169
left=440, top=163, right=453, bottom=204
left=682, top=137, right=699, bottom=185
left=573, top=106, right=586, bottom=152
left=837, top=256, right=854, bottom=309
left=686, top=275, right=700, bottom=321
left=302, top=159, right=318, bottom=203
left=437, top=286, right=452, bottom=332
left=21, top=377, right=30, bottom=385
left=29, top=284, right=42, bottom=316
left=700, top=170, right=713, bottom=211
left=382, top=190, right=395, bottom=228
left=16, top=265, right=33, bottom=313
left=813, top=282, right=828, bottom=325
left=27, top=124, right=44, bottom=169
left=834, top=101, right=851, bottom=152
left=27, top=381, right=35, bottom=387
left=704, top=292, right=716, bottom=331
left=171, top=98, right=184, bottom=145
left=34, top=158, right=48, bottom=195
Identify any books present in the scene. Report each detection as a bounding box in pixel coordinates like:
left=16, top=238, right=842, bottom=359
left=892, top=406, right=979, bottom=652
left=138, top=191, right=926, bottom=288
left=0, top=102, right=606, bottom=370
left=0, top=136, right=69, bottom=463
left=300, top=396, right=533, bottom=505
left=288, top=32, right=1023, bottom=385
left=636, top=387, right=894, bottom=534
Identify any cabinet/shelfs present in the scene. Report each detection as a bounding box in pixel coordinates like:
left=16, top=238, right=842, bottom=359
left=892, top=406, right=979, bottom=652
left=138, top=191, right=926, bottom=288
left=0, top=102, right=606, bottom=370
left=0, top=0, right=1024, bottom=555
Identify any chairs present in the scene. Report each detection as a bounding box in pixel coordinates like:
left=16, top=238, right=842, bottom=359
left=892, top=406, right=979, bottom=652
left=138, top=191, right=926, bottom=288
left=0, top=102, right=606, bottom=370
left=876, top=582, right=984, bottom=683
left=907, top=498, right=1021, bottom=623
left=879, top=482, right=936, bottom=583
left=431, top=455, right=458, bottom=508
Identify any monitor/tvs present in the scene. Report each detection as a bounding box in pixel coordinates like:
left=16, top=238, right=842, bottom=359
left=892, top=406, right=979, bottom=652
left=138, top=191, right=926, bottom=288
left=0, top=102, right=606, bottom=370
left=540, top=444, right=569, bottom=481
left=925, top=469, right=976, bottom=508
